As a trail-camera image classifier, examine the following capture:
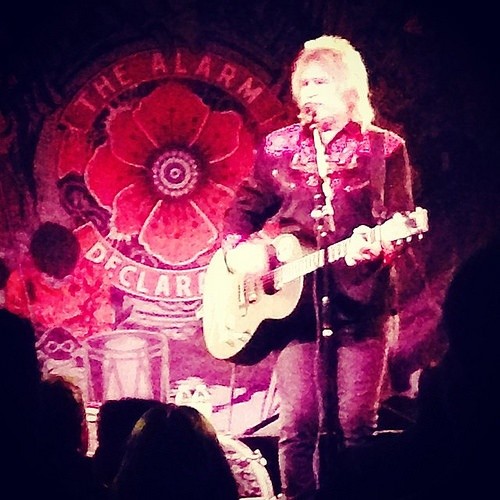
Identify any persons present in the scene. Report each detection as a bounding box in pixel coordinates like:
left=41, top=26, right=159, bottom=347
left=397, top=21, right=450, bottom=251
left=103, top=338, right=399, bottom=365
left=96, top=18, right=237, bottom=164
left=0, top=258, right=40, bottom=398
left=0, top=374, right=239, bottom=500
left=218, top=34, right=414, bottom=500
left=290, top=254, right=500, bottom=500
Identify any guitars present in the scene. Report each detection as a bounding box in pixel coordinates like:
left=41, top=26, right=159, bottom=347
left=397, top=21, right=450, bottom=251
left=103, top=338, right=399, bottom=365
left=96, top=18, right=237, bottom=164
left=202, top=207, right=431, bottom=365
